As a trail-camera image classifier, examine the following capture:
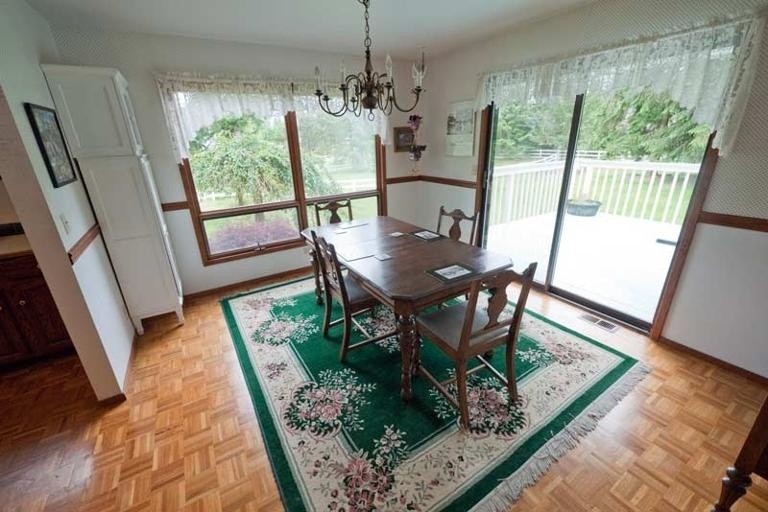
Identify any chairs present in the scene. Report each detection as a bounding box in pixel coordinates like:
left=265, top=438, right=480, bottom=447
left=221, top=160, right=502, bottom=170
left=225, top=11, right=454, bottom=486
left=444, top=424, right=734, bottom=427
left=411, top=262, right=538, bottom=428
left=437, top=206, right=480, bottom=310
left=314, top=198, right=353, bottom=226
left=311, top=230, right=403, bottom=363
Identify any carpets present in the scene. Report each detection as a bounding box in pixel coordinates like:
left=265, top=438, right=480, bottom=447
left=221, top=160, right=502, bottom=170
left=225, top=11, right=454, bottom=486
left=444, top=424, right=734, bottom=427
left=218, top=267, right=652, bottom=511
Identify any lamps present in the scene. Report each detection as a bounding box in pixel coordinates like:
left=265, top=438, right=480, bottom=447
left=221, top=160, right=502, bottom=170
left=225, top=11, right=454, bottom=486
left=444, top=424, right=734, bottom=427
left=314, top=0, right=427, bottom=118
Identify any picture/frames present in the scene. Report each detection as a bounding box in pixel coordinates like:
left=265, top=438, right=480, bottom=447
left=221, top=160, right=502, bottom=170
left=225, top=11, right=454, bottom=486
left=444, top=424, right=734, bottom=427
left=394, top=126, right=414, bottom=152
left=24, top=102, right=77, bottom=189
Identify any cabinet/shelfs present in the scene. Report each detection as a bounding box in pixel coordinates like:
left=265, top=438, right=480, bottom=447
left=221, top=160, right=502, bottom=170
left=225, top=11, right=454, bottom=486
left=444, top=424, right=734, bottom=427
left=41, top=63, right=143, bottom=158
left=0, top=256, right=73, bottom=370
left=77, top=155, right=186, bottom=335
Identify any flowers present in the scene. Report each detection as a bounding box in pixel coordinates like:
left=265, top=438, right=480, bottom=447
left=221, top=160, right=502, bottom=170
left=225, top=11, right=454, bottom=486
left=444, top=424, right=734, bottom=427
left=407, top=115, right=422, bottom=146
